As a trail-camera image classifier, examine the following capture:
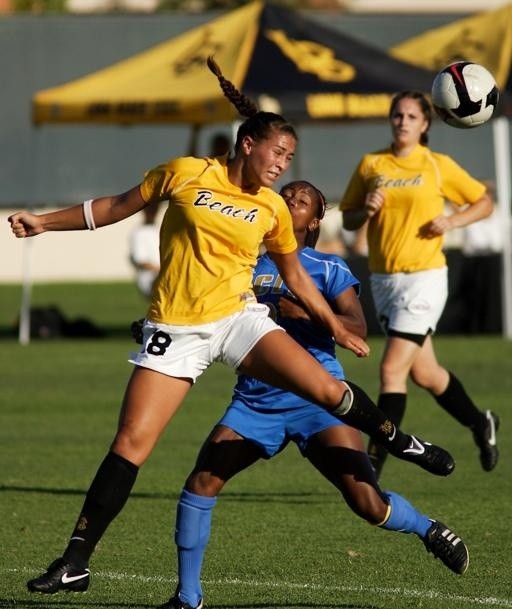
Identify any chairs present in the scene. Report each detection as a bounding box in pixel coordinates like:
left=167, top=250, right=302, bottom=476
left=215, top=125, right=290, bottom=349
left=393, top=435, right=456, bottom=476
left=27, top=557, right=89, bottom=593
left=419, top=519, right=469, bottom=575
left=475, top=409, right=499, bottom=471
left=158, top=595, right=203, bottom=609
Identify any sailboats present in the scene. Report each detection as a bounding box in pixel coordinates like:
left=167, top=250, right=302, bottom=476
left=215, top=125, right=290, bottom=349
left=432, top=60, right=499, bottom=129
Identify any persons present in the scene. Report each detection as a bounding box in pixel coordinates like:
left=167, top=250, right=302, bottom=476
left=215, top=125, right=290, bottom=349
left=314, top=180, right=502, bottom=261
left=154, top=178, right=473, bottom=609
left=334, top=88, right=502, bottom=488
left=126, top=203, right=165, bottom=303
left=4, top=52, right=460, bottom=595
left=208, top=130, right=233, bottom=159
